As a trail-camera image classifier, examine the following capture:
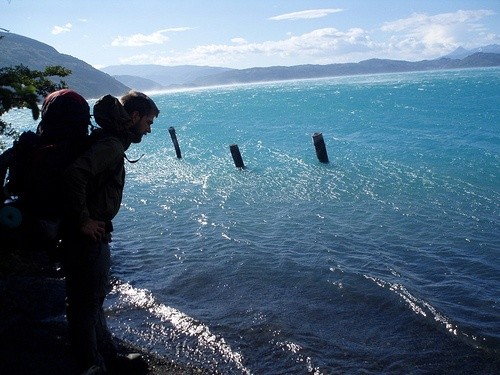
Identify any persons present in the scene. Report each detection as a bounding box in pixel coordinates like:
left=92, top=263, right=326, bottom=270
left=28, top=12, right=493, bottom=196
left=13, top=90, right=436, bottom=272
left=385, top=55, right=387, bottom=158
left=68, top=88, right=161, bottom=375
left=0, top=89, right=108, bottom=374
